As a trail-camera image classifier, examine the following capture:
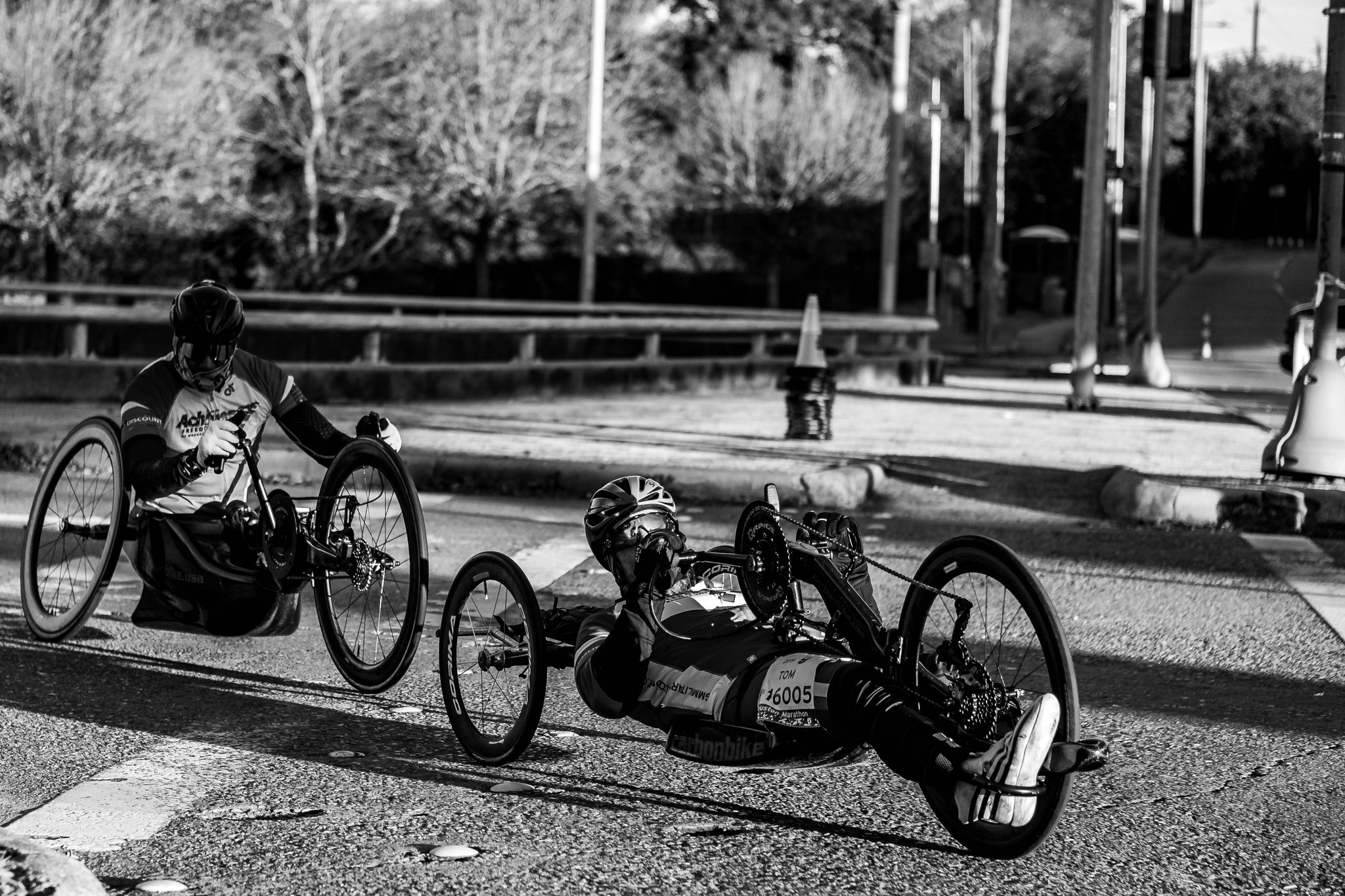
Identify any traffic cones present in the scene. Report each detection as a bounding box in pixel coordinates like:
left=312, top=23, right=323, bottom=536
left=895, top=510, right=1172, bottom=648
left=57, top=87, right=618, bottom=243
left=791, top=291, right=831, bottom=370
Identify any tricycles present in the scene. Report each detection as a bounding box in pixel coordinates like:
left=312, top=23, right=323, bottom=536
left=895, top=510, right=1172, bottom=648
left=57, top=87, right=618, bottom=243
left=435, top=482, right=1108, bottom=859
left=19, top=412, right=430, bottom=693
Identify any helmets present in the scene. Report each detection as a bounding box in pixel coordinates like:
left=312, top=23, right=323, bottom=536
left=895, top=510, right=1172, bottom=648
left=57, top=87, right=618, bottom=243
left=168, top=279, right=246, bottom=346
left=583, top=475, right=679, bottom=570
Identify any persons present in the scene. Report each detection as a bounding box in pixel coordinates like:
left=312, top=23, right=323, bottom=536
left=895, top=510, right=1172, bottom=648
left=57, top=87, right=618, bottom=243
left=120, top=281, right=401, bottom=637
left=573, top=475, right=1060, bottom=827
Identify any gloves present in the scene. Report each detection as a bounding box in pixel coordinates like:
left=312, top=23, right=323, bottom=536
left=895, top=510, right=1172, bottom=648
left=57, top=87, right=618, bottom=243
left=355, top=410, right=402, bottom=452
left=633, top=529, right=683, bottom=599
left=193, top=420, right=247, bottom=469
left=796, top=510, right=883, bottom=633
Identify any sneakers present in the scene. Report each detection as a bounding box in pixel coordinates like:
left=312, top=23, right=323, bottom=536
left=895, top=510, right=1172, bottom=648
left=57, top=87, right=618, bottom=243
left=953, top=692, right=1060, bottom=826
